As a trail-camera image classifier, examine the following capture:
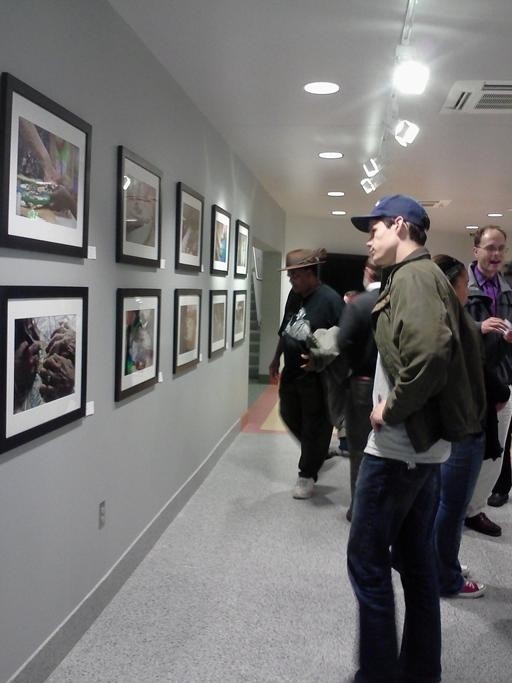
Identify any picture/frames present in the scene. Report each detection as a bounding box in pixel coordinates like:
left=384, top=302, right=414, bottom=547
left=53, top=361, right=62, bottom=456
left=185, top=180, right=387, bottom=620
left=114, top=288, right=161, bottom=403
left=209, top=289, right=228, bottom=359
left=234, top=219, right=249, bottom=277
left=175, top=181, right=205, bottom=272
left=0, top=285, right=89, bottom=455
left=174, top=289, right=203, bottom=374
left=116, top=145, right=164, bottom=268
left=232, top=290, right=247, bottom=347
left=211, top=204, right=231, bottom=275
left=0, top=72, right=92, bottom=258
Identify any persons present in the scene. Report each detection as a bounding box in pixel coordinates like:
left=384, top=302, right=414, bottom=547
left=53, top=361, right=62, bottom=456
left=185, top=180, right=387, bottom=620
left=270, top=247, right=344, bottom=498
left=465, top=225, right=512, bottom=536
left=345, top=195, right=485, bottom=681
left=16, top=320, right=77, bottom=403
left=431, top=255, right=486, bottom=599
left=327, top=257, right=382, bottom=522
left=19, top=118, right=77, bottom=221
left=339, top=291, right=359, bottom=450
left=488, top=416, right=512, bottom=506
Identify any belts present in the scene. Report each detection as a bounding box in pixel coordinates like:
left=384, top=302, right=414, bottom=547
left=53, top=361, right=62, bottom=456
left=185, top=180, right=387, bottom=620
left=350, top=374, right=372, bottom=385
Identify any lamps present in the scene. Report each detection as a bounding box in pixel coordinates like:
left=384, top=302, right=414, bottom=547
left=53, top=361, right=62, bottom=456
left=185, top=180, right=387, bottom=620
left=360, top=46, right=430, bottom=196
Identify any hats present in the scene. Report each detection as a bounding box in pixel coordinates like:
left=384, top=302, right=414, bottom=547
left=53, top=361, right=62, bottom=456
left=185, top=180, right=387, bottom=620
left=277, top=248, right=326, bottom=271
left=350, top=193, right=429, bottom=234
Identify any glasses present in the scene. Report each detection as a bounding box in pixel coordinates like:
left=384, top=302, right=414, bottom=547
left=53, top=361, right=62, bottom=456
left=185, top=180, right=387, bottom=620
left=475, top=245, right=509, bottom=252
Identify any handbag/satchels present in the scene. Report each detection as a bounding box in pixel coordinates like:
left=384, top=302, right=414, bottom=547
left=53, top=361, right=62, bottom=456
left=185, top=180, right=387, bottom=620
left=282, top=333, right=309, bottom=370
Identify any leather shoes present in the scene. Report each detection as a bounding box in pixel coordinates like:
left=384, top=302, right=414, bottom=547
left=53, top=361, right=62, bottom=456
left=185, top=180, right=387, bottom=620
left=487, top=491, right=509, bottom=507
left=464, top=510, right=502, bottom=537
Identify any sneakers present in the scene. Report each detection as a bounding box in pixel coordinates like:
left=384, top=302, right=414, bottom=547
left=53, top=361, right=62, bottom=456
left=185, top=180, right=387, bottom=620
left=456, top=578, right=487, bottom=599
left=291, top=475, right=315, bottom=500
left=335, top=437, right=352, bottom=457
left=460, top=564, right=472, bottom=577
